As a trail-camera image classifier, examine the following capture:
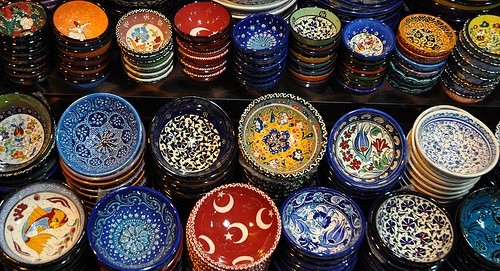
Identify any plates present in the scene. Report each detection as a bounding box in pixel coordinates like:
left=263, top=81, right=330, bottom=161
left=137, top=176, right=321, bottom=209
left=0, top=85, right=500, bottom=271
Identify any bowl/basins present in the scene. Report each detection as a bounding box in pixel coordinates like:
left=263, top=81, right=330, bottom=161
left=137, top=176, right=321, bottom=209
left=1, top=0, right=500, bottom=110
